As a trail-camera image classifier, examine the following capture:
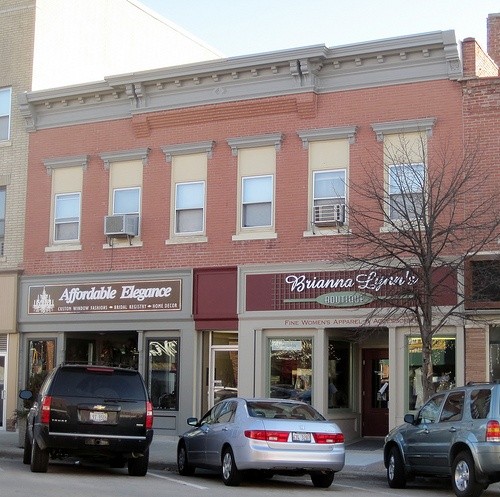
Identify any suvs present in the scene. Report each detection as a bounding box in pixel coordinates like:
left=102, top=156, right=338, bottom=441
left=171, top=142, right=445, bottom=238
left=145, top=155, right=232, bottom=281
left=18, top=360, right=155, bottom=476
left=383, top=381, right=500, bottom=497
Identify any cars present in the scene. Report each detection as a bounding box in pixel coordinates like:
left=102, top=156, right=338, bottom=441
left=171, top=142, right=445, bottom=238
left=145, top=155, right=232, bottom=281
left=177, top=397, right=346, bottom=488
left=159, top=382, right=312, bottom=411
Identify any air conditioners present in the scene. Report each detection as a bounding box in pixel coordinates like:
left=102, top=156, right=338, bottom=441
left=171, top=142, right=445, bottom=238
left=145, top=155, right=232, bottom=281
left=103, top=215, right=137, bottom=237
